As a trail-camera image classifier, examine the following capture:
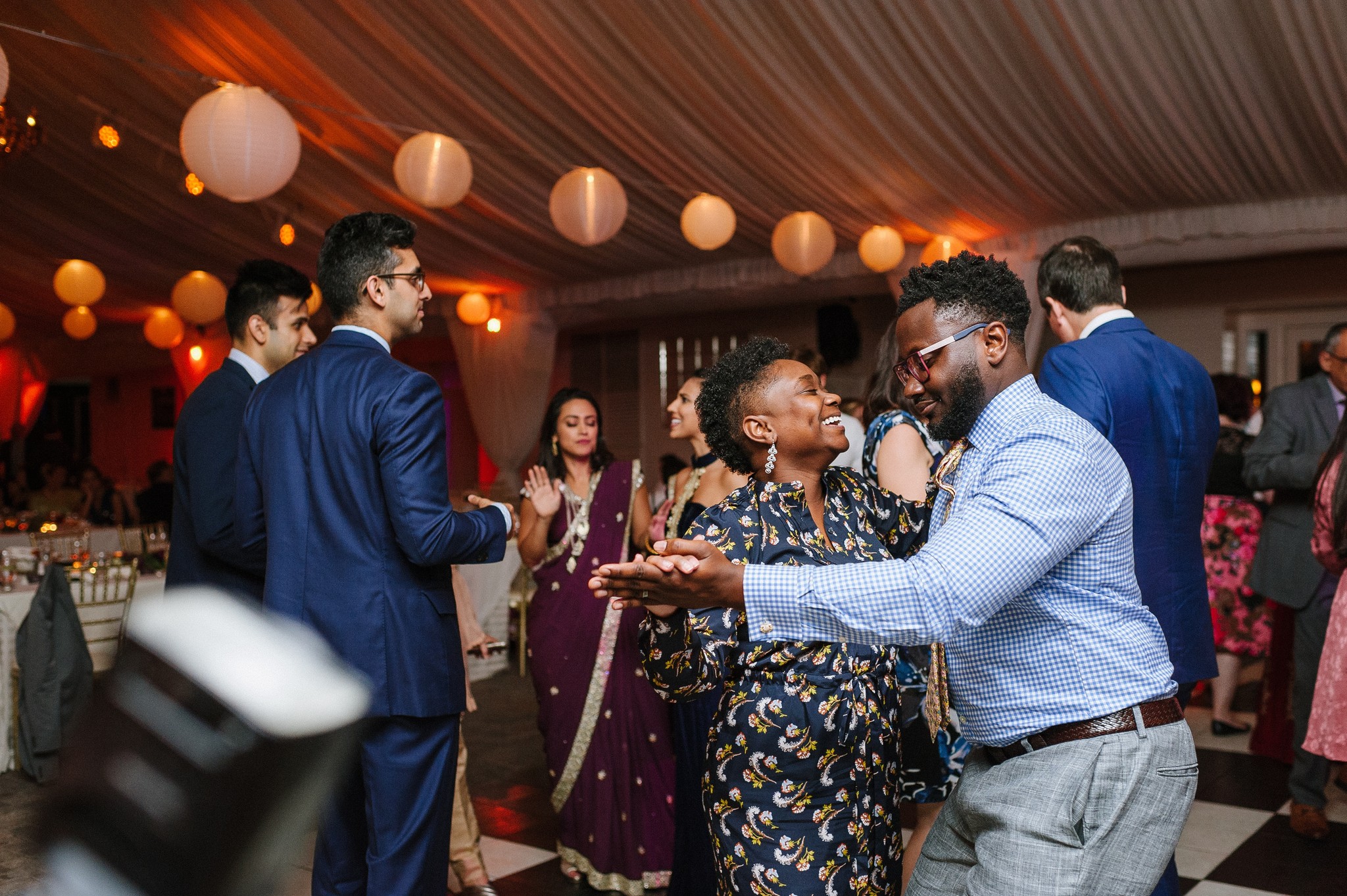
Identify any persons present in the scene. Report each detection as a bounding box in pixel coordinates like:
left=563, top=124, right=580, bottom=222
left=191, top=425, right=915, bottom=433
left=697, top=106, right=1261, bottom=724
left=859, top=319, right=980, bottom=896
left=585, top=249, right=1202, bottom=896
left=162, top=256, right=320, bottom=617
left=1200, top=373, right=1280, bottom=735
left=1242, top=321, right=1346, bottom=850
left=1299, top=402, right=1346, bottom=774
left=234, top=211, right=523, bottom=895
left=0, top=421, right=172, bottom=562
left=633, top=333, right=958, bottom=896
left=778, top=346, right=871, bottom=484
left=1024, top=235, right=1227, bottom=896
left=516, top=386, right=683, bottom=895
left=442, top=491, right=508, bottom=894
left=642, top=369, right=756, bottom=896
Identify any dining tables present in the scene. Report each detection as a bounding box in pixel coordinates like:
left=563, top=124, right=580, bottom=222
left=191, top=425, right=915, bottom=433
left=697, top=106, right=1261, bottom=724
left=2, top=569, right=170, bottom=693
left=0, top=522, right=149, bottom=576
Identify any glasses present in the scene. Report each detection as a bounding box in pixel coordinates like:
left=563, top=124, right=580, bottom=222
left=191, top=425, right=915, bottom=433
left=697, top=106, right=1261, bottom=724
left=891, top=323, right=1010, bottom=387
left=362, top=272, right=425, bottom=295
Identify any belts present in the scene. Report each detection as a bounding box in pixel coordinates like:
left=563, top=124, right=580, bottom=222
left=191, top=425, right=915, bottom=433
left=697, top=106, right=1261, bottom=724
left=983, top=697, right=1186, bottom=766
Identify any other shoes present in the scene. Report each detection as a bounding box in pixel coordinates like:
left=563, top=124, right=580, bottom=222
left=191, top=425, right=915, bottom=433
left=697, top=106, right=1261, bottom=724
left=1211, top=718, right=1251, bottom=736
left=1334, top=768, right=1346, bottom=796
left=464, top=883, right=499, bottom=896
left=1290, top=803, right=1327, bottom=841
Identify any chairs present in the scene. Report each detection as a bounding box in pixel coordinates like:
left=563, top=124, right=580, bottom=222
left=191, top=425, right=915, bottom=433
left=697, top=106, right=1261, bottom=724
left=9, top=565, right=141, bottom=772
left=116, top=522, right=154, bottom=564
left=24, top=528, right=91, bottom=563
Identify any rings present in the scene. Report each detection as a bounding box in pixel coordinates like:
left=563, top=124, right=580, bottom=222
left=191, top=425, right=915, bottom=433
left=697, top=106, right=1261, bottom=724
left=642, top=590, right=649, bottom=599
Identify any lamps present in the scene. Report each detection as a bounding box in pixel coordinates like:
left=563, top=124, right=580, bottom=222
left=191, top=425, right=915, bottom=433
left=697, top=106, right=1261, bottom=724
left=0, top=45, right=983, bottom=359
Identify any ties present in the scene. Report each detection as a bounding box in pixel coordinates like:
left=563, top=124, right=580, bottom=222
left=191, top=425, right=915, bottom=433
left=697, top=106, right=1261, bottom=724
left=930, top=436, right=969, bottom=525
